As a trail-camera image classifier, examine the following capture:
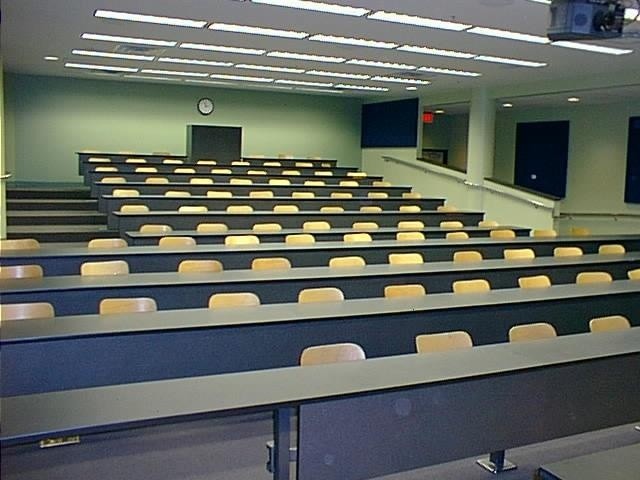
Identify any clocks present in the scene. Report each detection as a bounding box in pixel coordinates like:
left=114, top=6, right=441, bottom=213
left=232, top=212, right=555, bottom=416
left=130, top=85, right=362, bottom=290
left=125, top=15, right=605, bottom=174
left=197, top=97, right=215, bottom=115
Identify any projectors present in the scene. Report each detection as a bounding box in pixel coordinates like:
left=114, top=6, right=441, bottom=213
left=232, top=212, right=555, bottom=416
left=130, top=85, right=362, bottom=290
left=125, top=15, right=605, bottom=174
left=547, top=0, right=624, bottom=35
